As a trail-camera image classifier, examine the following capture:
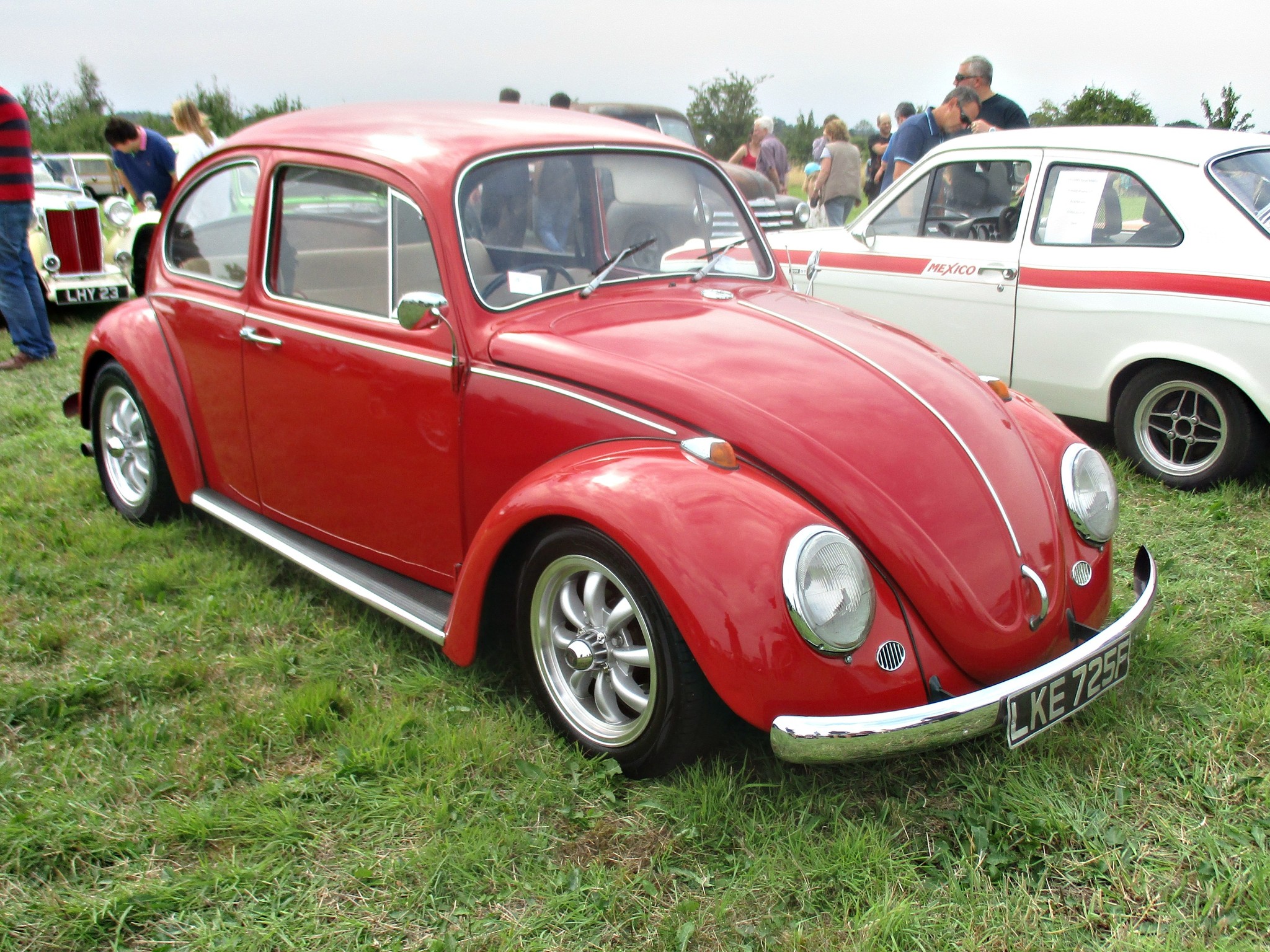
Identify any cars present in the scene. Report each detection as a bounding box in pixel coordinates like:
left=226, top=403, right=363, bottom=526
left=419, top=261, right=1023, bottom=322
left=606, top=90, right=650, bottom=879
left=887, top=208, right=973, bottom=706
left=58, top=98, right=1160, bottom=782
left=528, top=99, right=813, bottom=270
left=25, top=151, right=163, bottom=317
left=650, top=117, right=1270, bottom=493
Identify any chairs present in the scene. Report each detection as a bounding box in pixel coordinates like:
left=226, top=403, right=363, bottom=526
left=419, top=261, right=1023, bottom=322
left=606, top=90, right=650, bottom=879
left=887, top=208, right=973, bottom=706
left=476, top=267, right=593, bottom=307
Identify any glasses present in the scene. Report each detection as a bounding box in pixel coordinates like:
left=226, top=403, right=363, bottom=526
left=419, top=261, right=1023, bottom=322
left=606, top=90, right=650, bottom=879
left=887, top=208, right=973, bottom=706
left=957, top=100, right=972, bottom=125
left=955, top=73, right=984, bottom=82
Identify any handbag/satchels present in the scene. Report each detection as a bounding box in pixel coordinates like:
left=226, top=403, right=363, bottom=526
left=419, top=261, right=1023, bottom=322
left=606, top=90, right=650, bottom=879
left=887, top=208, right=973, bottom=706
left=864, top=181, right=873, bottom=195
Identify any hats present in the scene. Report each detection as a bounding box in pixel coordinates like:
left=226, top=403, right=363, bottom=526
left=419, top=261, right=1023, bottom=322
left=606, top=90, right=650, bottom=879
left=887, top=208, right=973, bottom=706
left=804, top=162, right=821, bottom=176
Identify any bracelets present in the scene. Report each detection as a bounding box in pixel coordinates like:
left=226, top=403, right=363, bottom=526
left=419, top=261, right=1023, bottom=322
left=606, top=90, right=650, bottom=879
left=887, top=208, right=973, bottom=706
left=814, top=188, right=819, bottom=192
left=989, top=127, right=996, bottom=133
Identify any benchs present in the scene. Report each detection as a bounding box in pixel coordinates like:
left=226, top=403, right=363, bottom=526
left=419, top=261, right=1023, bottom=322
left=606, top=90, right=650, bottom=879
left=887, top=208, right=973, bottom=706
left=1121, top=215, right=1176, bottom=246
left=179, top=238, right=496, bottom=320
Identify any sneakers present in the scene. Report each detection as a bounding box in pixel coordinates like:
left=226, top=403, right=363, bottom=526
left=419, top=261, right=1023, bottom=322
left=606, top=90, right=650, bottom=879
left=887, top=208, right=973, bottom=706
left=0, top=350, right=56, bottom=371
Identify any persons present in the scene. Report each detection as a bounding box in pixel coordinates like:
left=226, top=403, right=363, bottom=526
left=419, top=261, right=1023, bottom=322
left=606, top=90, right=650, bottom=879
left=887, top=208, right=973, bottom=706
left=863, top=101, right=915, bottom=206
left=0, top=87, right=58, bottom=371
left=470, top=89, right=529, bottom=249
left=879, top=85, right=981, bottom=219
left=945, top=54, right=1032, bottom=218
left=103, top=118, right=178, bottom=212
left=170, top=97, right=221, bottom=181
left=534, top=92, right=577, bottom=253
left=726, top=115, right=789, bottom=195
left=802, top=114, right=862, bottom=227
